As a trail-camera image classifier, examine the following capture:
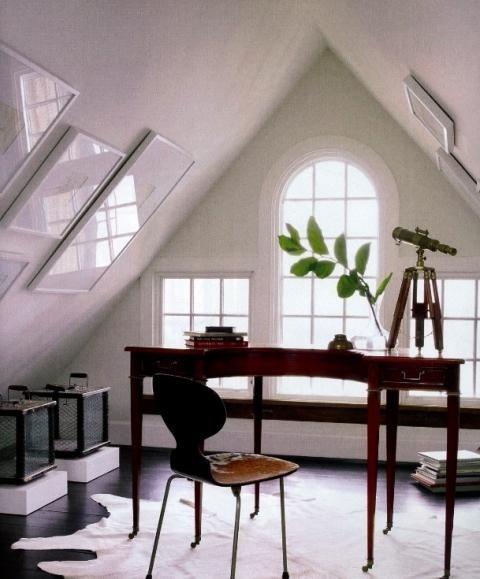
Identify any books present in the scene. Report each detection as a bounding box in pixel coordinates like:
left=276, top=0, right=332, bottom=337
left=409, top=448, right=480, bottom=494
left=183, top=325, right=249, bottom=347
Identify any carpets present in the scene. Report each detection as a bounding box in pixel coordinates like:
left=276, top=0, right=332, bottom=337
left=10, top=485, right=480, bottom=579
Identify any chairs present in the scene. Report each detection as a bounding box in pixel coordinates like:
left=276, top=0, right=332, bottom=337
left=143, top=373, right=301, bottom=579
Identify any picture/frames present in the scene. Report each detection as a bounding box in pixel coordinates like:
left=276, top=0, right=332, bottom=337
left=0, top=40, right=78, bottom=199
left=1, top=252, right=30, bottom=301
left=29, top=131, right=194, bottom=295
left=1, top=129, right=128, bottom=239
left=401, top=74, right=479, bottom=210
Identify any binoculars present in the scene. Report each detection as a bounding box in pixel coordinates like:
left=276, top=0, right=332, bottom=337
left=392, top=227, right=457, bottom=258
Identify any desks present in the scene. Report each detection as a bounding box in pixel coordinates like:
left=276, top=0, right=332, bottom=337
left=123, top=344, right=465, bottom=579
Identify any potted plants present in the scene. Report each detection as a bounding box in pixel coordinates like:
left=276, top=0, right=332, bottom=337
left=276, top=215, right=399, bottom=352
